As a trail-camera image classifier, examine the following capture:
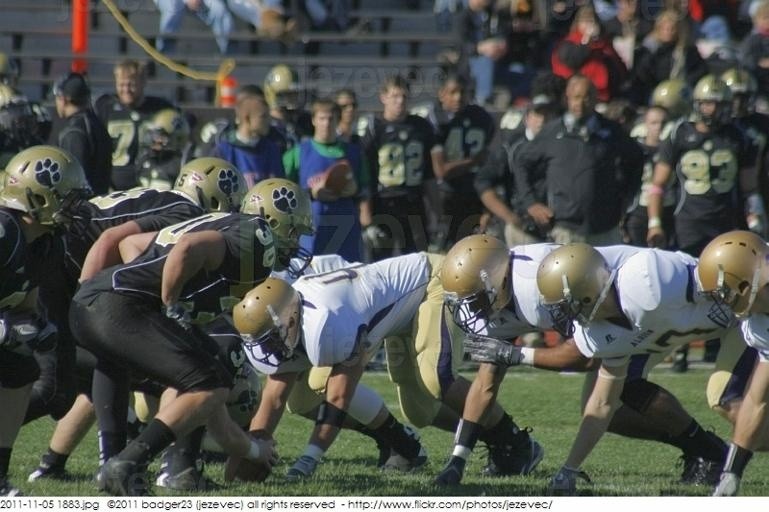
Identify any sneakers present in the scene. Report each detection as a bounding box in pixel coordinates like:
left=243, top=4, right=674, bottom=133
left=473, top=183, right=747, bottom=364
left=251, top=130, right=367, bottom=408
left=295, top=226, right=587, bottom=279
left=155, top=447, right=226, bottom=491
left=89, top=454, right=156, bottom=497
left=670, top=351, right=690, bottom=373
left=28, top=455, right=72, bottom=483
left=375, top=440, right=428, bottom=473
left=683, top=438, right=731, bottom=487
left=471, top=441, right=502, bottom=476
left=674, top=452, right=700, bottom=481
left=498, top=425, right=545, bottom=476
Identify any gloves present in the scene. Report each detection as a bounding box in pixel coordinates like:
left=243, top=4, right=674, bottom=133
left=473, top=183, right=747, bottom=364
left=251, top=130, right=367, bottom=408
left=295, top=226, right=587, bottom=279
left=3, top=308, right=57, bottom=353
left=648, top=223, right=669, bottom=249
left=360, top=224, right=386, bottom=253
left=462, top=330, right=520, bottom=368
left=166, top=300, right=220, bottom=355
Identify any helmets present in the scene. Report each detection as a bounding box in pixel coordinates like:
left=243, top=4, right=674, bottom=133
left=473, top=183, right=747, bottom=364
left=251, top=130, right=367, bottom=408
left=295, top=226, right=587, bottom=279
left=137, top=108, right=190, bottom=159
left=264, top=63, right=305, bottom=115
left=721, top=66, right=759, bottom=116
left=648, top=77, right=693, bottom=119
left=231, top=279, right=303, bottom=366
left=241, top=176, right=317, bottom=276
left=695, top=229, right=769, bottom=330
left=175, top=156, right=249, bottom=214
left=535, top=241, right=610, bottom=338
left=0, top=52, right=18, bottom=85
left=0, top=143, right=94, bottom=243
left=440, top=233, right=513, bottom=335
left=692, top=75, right=735, bottom=129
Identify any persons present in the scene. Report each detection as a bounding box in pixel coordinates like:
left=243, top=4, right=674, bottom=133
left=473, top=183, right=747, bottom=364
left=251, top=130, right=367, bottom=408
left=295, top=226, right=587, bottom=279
left=514, top=73, right=642, bottom=247
left=205, top=95, right=293, bottom=190
left=364, top=74, right=446, bottom=264
left=649, top=75, right=762, bottom=373
left=459, top=0, right=637, bottom=115
left=71, top=179, right=317, bottom=496
left=331, top=88, right=360, bottom=143
left=225, top=0, right=313, bottom=46
left=27, top=157, right=249, bottom=483
left=262, top=65, right=301, bottom=122
left=48, top=72, right=113, bottom=196
left=283, top=99, right=362, bottom=263
left=463, top=243, right=757, bottom=496
left=438, top=233, right=728, bottom=497
left=0, top=50, right=52, bottom=171
left=698, top=230, right=769, bottom=496
left=233, top=251, right=543, bottom=482
left=0, top=145, right=92, bottom=496
left=473, top=103, right=557, bottom=248
left=267, top=254, right=426, bottom=471
left=192, top=86, right=293, bottom=167
left=627, top=108, right=672, bottom=244
left=427, top=70, right=496, bottom=250
left=637, top=0, right=768, bottom=106
left=93, top=59, right=192, bottom=193
left=152, top=0, right=233, bottom=53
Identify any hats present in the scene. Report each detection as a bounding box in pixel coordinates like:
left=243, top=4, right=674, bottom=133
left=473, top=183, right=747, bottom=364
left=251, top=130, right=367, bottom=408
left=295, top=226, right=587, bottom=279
left=530, top=92, right=555, bottom=110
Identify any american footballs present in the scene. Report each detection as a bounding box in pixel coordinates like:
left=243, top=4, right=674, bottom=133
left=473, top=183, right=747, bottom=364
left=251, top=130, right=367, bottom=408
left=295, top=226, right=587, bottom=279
left=224, top=429, right=276, bottom=484
